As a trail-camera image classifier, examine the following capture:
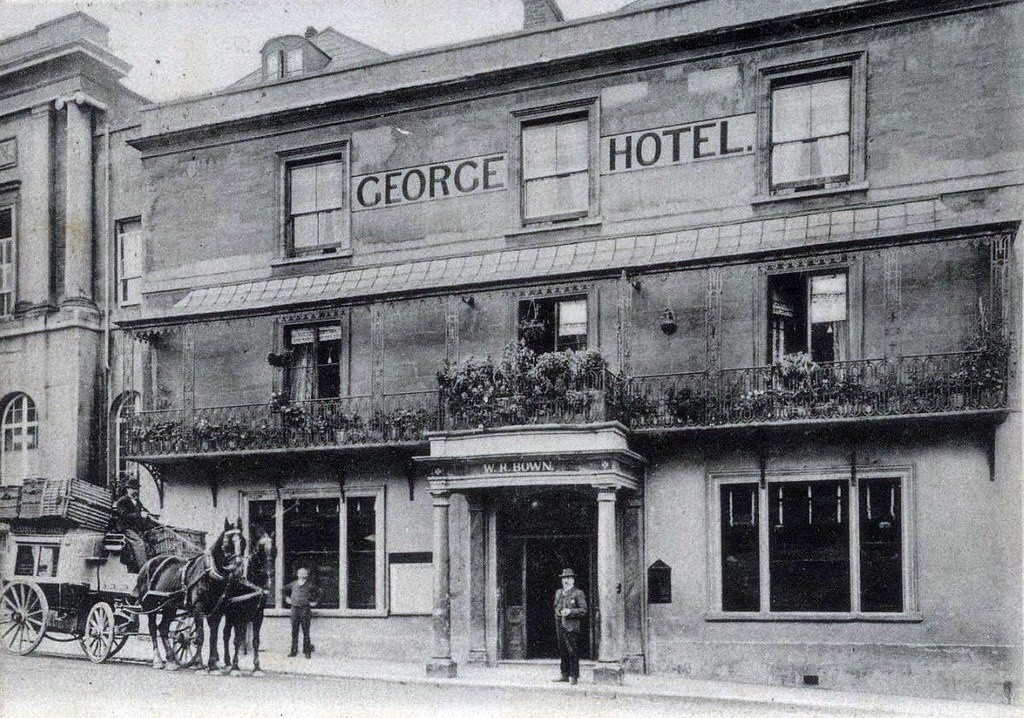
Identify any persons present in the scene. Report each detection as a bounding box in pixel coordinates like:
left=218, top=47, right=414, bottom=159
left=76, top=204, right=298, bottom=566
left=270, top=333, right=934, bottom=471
left=116, top=478, right=148, bottom=569
left=281, top=568, right=324, bottom=659
left=551, top=568, right=588, bottom=685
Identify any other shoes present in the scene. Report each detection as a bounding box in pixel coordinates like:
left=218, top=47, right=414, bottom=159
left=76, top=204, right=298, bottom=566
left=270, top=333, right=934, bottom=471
left=305, top=653, right=311, bottom=658
left=288, top=652, right=297, bottom=656
left=553, top=674, right=569, bottom=682
left=571, top=677, right=578, bottom=685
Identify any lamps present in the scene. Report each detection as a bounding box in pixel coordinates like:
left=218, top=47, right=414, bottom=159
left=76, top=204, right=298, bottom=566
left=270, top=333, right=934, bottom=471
left=621, top=269, right=641, bottom=293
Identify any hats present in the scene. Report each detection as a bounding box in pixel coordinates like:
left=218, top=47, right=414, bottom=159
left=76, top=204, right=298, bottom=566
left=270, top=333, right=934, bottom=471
left=559, top=569, right=577, bottom=577
left=124, top=480, right=142, bottom=488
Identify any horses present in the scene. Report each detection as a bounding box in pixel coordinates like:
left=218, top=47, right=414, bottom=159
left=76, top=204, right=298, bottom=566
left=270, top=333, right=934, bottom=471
left=138, top=517, right=277, bottom=678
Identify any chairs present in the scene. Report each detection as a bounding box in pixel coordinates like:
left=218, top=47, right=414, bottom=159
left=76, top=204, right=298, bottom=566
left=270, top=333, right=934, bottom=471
left=102, top=478, right=126, bottom=552
